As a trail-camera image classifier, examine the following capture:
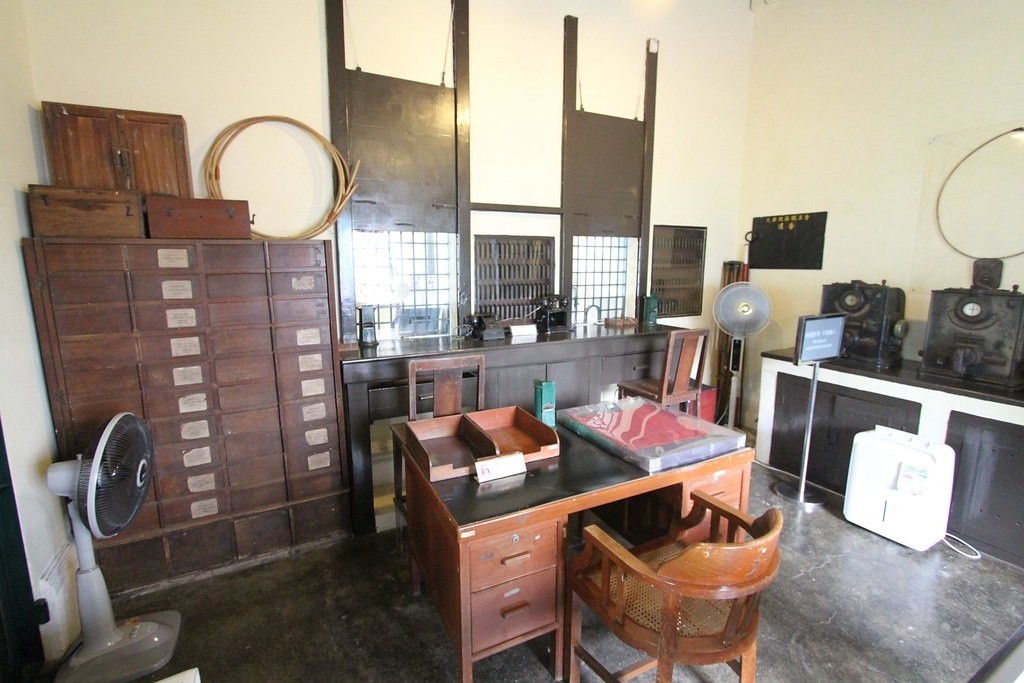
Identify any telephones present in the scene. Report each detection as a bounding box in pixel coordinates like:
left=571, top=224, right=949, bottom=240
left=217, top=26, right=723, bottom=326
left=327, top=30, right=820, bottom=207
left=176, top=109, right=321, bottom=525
left=470, top=312, right=507, bottom=341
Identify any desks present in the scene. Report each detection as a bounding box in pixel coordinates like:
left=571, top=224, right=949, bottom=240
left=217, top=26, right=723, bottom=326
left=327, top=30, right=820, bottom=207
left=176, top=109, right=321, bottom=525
left=337, top=323, right=693, bottom=495
left=404, top=396, right=756, bottom=683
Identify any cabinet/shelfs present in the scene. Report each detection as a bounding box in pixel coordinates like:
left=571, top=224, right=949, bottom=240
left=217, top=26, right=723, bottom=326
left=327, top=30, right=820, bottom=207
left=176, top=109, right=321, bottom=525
left=943, top=409, right=1024, bottom=572
left=770, top=369, right=923, bottom=497
left=41, top=101, right=193, bottom=201
left=19, top=239, right=352, bottom=597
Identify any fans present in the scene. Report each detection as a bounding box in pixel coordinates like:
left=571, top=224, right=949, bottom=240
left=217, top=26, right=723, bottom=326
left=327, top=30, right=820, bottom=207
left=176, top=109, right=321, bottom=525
left=46, top=410, right=181, bottom=683
left=713, top=281, right=774, bottom=447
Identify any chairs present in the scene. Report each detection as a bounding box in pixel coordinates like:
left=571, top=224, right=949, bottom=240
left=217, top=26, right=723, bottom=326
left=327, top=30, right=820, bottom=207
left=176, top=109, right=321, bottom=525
left=389, top=354, right=484, bottom=498
left=616, top=327, right=710, bottom=419
left=561, top=490, right=783, bottom=683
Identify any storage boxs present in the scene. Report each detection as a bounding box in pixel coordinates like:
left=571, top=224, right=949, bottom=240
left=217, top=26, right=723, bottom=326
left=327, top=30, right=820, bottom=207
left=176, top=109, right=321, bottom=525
left=144, top=195, right=254, bottom=239
left=28, top=183, right=146, bottom=241
